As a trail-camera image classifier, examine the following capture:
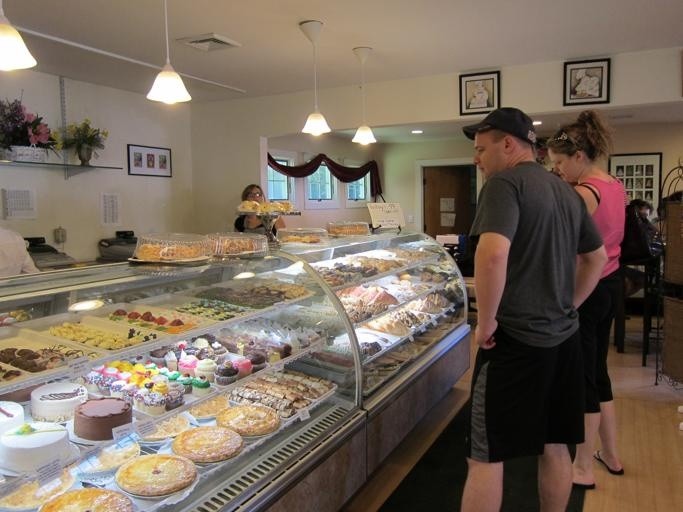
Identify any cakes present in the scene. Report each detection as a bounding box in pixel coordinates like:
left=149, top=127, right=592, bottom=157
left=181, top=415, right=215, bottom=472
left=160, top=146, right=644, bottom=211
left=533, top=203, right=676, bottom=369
left=31, top=381, right=88, bottom=422
left=1, top=422, right=70, bottom=475
left=74, top=397, right=132, bottom=441
left=1, top=401, right=24, bottom=432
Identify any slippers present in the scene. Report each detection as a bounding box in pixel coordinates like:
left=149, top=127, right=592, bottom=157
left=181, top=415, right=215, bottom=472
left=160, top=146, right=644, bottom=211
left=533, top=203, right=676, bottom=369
left=592, top=450, right=625, bottom=472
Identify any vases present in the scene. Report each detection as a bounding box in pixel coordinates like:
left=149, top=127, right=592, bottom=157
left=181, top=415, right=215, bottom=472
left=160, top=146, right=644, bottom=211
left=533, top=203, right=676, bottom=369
left=0, top=144, right=48, bottom=163
left=78, top=142, right=94, bottom=167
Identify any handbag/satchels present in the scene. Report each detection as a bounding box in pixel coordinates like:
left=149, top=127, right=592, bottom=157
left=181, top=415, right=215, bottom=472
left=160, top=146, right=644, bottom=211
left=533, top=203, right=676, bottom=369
left=619, top=205, right=666, bottom=264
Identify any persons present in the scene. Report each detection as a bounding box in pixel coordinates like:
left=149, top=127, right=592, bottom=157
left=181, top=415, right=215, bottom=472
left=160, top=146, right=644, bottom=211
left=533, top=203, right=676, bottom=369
left=458, top=103, right=610, bottom=512
left=622, top=197, right=664, bottom=330
left=231, top=184, right=284, bottom=236
left=0, top=226, right=40, bottom=279
left=541, top=116, right=630, bottom=491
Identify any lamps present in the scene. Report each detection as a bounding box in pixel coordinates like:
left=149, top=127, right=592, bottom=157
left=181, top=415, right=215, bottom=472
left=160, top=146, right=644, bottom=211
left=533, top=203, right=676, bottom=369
left=296, top=18, right=332, bottom=138
left=350, top=46, right=378, bottom=147
left=0, top=1, right=38, bottom=73
left=144, top=1, right=192, bottom=107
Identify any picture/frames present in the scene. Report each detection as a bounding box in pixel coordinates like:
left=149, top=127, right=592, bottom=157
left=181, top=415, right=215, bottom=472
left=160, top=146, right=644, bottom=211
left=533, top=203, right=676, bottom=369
left=561, top=56, right=611, bottom=109
left=607, top=151, right=663, bottom=222
left=125, top=143, right=173, bottom=178
left=456, top=69, right=501, bottom=117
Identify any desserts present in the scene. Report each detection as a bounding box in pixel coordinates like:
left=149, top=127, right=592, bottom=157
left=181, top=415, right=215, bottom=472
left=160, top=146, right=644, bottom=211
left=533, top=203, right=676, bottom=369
left=273, top=243, right=451, bottom=395
left=228, top=370, right=333, bottom=418
left=138, top=243, right=201, bottom=262
left=222, top=238, right=257, bottom=253
left=285, top=236, right=320, bottom=243
left=328, top=225, right=368, bottom=235
left=0, top=279, right=306, bottom=414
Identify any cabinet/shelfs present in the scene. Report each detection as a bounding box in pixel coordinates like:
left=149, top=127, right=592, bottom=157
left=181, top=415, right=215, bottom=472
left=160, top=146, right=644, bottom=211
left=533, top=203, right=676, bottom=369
left=279, top=230, right=472, bottom=511
left=0, top=246, right=368, bottom=511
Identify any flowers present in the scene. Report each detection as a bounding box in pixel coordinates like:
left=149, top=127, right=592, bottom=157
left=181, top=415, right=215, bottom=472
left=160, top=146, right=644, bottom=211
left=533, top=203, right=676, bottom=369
left=48, top=116, right=110, bottom=152
left=0, top=88, right=62, bottom=160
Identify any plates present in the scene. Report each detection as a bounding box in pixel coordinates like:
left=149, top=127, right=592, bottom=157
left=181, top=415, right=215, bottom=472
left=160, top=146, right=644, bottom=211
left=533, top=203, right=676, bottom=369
left=0, top=393, right=280, bottom=510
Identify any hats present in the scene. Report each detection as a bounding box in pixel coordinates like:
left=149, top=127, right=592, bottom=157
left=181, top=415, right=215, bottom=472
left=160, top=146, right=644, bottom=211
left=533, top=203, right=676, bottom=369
left=462, top=106, right=536, bottom=145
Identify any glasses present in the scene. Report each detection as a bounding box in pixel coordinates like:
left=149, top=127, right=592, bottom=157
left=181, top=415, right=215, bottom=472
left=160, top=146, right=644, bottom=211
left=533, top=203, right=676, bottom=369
left=247, top=192, right=263, bottom=198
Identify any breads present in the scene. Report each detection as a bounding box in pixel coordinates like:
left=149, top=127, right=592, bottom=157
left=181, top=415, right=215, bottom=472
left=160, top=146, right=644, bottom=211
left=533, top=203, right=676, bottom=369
left=38, top=488, right=132, bottom=512
left=172, top=426, right=242, bottom=462
left=237, top=200, right=294, bottom=213
left=188, top=396, right=228, bottom=417
left=2, top=470, right=72, bottom=507
left=78, top=439, right=138, bottom=474
left=216, top=405, right=279, bottom=434
left=117, top=454, right=196, bottom=495
left=141, top=414, right=190, bottom=441
